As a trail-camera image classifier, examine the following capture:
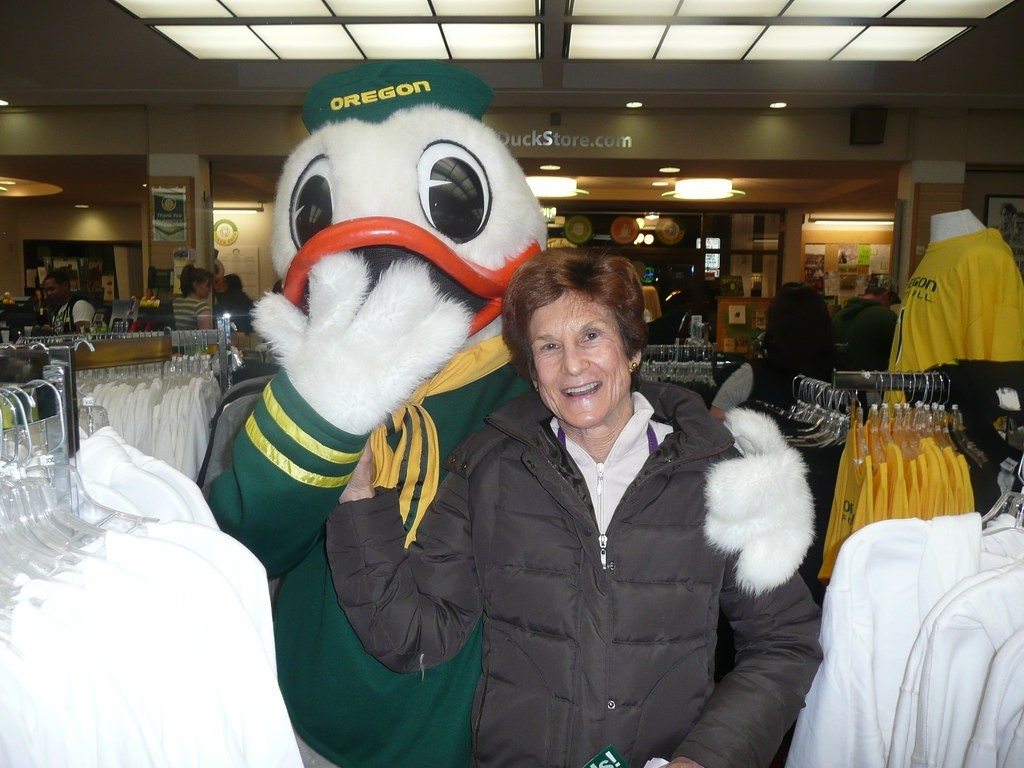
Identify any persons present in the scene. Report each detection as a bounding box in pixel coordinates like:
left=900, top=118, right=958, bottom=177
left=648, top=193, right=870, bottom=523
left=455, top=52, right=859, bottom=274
left=0, top=264, right=255, bottom=335
left=831, top=272, right=902, bottom=371
left=710, top=362, right=777, bottom=423
left=326, top=245, right=824, bottom=768
left=766, top=281, right=831, bottom=382
left=888, top=208, right=1024, bottom=374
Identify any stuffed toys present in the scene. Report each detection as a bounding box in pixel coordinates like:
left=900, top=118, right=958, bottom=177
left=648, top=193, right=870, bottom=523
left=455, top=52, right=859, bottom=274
left=205, top=59, right=548, bottom=768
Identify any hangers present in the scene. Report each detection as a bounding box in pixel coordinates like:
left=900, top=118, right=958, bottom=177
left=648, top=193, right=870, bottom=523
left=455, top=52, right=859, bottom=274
left=637, top=345, right=715, bottom=388
left=762, top=368, right=1024, bottom=529
left=0, top=327, right=222, bottom=626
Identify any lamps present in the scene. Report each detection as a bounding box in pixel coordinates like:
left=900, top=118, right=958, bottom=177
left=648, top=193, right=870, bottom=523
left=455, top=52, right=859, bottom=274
left=213, top=202, right=265, bottom=212
left=674, top=178, right=733, bottom=200
left=525, top=175, right=577, bottom=198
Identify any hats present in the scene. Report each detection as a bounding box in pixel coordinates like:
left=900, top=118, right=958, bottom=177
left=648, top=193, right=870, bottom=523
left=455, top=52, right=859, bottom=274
left=868, top=275, right=901, bottom=304
